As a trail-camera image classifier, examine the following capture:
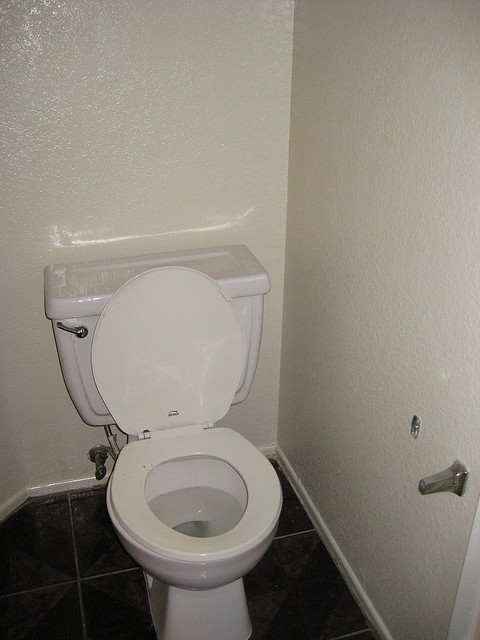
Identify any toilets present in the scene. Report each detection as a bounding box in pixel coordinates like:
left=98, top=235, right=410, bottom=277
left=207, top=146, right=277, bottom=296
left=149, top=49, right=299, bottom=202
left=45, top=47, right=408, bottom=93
left=41, top=243, right=285, bottom=639
